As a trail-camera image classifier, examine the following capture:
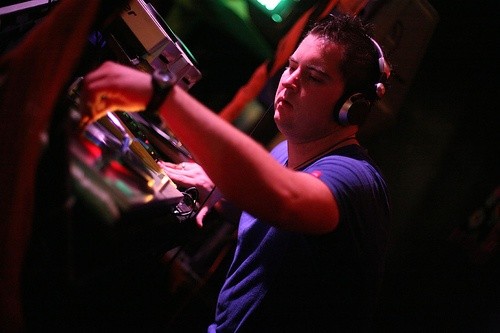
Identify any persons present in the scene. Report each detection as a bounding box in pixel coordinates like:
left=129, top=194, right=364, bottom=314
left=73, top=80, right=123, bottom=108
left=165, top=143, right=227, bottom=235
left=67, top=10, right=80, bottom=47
left=77, top=11, right=392, bottom=333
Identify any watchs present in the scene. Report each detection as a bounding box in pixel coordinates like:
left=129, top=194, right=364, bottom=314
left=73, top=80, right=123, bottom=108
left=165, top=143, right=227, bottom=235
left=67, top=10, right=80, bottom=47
left=144, top=67, right=176, bottom=116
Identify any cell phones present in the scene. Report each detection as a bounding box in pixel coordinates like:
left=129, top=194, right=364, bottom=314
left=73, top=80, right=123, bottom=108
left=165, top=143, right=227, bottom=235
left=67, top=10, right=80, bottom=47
left=122, top=1, right=203, bottom=90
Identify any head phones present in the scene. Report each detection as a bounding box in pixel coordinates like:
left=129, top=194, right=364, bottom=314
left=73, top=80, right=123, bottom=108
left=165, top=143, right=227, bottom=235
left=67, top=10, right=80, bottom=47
left=335, top=34, right=391, bottom=127
